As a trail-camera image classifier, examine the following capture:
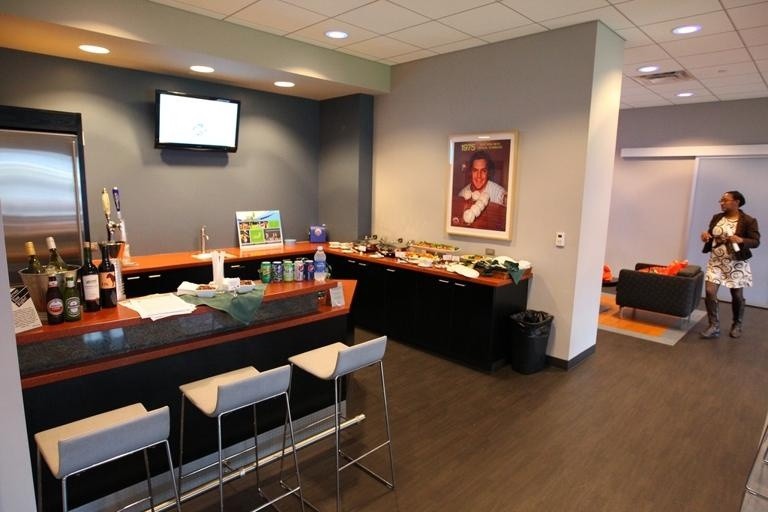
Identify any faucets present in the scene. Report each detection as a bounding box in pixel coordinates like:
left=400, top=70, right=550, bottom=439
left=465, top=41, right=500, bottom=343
left=199, top=227, right=210, bottom=253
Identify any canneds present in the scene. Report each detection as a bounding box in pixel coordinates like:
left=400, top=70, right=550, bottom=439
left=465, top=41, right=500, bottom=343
left=260, top=260, right=315, bottom=284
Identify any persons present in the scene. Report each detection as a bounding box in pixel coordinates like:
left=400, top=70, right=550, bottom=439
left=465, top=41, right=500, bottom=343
left=700, top=190, right=761, bottom=338
left=457, top=148, right=507, bottom=209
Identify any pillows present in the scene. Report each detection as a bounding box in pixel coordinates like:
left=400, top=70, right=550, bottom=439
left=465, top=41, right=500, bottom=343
left=635, top=259, right=689, bottom=277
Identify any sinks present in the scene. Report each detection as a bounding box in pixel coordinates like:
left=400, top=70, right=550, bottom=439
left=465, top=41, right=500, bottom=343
left=190, top=250, right=236, bottom=261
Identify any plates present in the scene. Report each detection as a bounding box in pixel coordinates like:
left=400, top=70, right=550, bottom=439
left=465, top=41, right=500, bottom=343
left=464, top=254, right=481, bottom=263
left=408, top=242, right=462, bottom=254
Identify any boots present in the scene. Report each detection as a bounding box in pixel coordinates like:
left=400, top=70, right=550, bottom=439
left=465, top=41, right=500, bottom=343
left=700, top=300, right=720, bottom=337
left=730, top=299, right=745, bottom=338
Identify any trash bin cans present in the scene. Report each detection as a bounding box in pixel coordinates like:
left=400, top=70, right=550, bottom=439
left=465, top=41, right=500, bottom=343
left=510, top=310, right=553, bottom=375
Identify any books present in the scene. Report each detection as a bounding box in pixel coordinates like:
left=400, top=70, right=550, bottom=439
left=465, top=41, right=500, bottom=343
left=118, top=291, right=198, bottom=323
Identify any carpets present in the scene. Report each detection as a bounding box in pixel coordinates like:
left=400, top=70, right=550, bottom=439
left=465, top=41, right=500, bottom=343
left=599, top=291, right=706, bottom=345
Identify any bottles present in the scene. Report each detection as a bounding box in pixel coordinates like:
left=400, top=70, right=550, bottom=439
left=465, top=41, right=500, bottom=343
left=44, top=274, right=65, bottom=326
left=43, top=237, right=67, bottom=274
left=100, top=244, right=117, bottom=305
left=23, top=239, right=43, bottom=274
left=313, top=246, right=326, bottom=281
left=65, top=273, right=80, bottom=324
left=80, top=239, right=100, bottom=311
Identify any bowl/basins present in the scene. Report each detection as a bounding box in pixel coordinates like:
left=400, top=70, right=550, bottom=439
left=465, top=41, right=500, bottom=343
left=284, top=239, right=296, bottom=245
left=236, top=279, right=256, bottom=294
left=196, top=285, right=216, bottom=297
left=328, top=241, right=353, bottom=249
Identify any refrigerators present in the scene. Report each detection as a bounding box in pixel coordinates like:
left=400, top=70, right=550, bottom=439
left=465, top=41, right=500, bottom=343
left=0, top=128, right=85, bottom=288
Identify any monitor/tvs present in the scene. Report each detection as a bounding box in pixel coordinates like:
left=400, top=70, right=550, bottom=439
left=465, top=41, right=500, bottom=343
left=154, top=87, right=241, bottom=152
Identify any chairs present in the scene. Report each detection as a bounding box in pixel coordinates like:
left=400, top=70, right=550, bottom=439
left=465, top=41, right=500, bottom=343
left=279, top=336, right=396, bottom=511
left=615, top=258, right=705, bottom=325
left=179, top=362, right=305, bottom=512
left=32, top=399, right=181, bottom=511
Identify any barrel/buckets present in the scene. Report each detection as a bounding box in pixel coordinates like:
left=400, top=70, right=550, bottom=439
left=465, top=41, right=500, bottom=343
left=17, top=262, right=81, bottom=315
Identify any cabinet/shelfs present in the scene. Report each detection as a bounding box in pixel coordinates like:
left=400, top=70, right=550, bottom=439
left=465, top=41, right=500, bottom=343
left=324, top=251, right=380, bottom=346
left=379, top=262, right=409, bottom=351
left=410, top=266, right=529, bottom=374
left=224, top=252, right=315, bottom=282
left=121, top=266, right=211, bottom=299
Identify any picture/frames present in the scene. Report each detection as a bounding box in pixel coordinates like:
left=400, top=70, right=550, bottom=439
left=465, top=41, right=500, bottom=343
left=445, top=129, right=520, bottom=245
